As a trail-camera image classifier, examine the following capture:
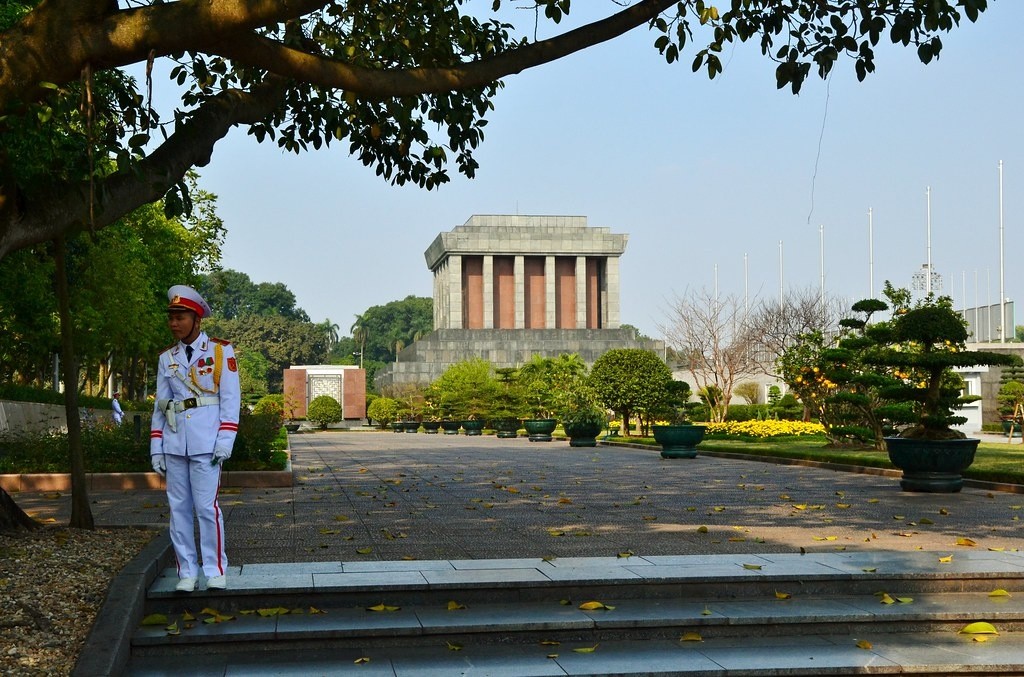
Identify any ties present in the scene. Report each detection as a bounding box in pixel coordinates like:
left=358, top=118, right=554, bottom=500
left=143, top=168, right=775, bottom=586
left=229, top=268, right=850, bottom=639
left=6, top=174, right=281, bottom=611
left=186, top=345, right=194, bottom=363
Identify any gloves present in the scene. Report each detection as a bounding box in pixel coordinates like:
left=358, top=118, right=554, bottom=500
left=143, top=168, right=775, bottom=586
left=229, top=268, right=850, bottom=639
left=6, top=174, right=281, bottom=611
left=121, top=412, right=125, bottom=418
left=151, top=454, right=166, bottom=477
left=212, top=447, right=231, bottom=464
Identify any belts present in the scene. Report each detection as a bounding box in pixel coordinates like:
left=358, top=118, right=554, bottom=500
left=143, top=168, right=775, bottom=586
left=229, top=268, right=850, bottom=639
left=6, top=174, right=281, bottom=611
left=174, top=396, right=220, bottom=412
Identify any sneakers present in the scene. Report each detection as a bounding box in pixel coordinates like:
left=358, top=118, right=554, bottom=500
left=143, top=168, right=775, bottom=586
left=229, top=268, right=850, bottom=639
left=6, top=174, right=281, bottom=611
left=175, top=575, right=199, bottom=591
left=206, top=574, right=226, bottom=588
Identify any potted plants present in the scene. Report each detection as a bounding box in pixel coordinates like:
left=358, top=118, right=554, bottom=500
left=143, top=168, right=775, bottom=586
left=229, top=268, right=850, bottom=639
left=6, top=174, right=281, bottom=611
left=277, top=387, right=301, bottom=434
left=860, top=309, right=1024, bottom=493
left=998, top=381, right=1024, bottom=437
left=522, top=406, right=557, bottom=441
left=391, top=409, right=521, bottom=438
left=560, top=409, right=602, bottom=447
left=650, top=381, right=707, bottom=458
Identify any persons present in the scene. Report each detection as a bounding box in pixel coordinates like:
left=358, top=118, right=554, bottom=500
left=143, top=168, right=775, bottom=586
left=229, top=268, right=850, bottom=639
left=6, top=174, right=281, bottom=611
left=111, top=391, right=124, bottom=425
left=150, top=284, right=240, bottom=592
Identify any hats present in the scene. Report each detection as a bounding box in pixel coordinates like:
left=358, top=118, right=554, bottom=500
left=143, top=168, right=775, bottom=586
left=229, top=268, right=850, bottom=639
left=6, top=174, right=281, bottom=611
left=167, top=285, right=211, bottom=321
left=112, top=392, right=121, bottom=397
